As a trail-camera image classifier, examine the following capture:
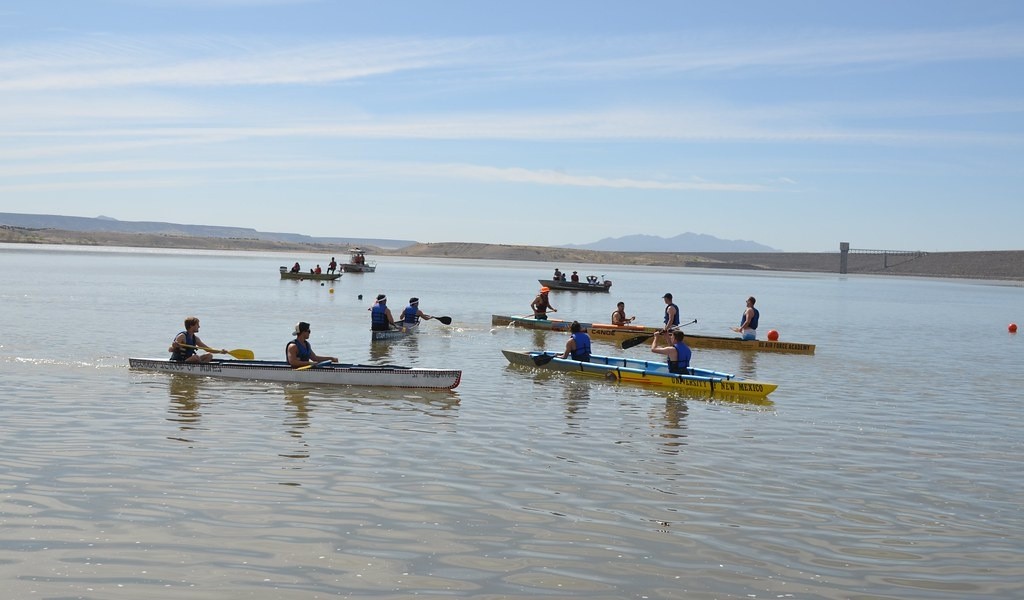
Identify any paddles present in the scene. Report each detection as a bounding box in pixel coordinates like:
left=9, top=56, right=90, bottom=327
left=622, top=318, right=697, bottom=349
left=619, top=317, right=634, bottom=326
left=730, top=326, right=741, bottom=331
left=374, top=299, right=406, bottom=333
left=173, top=342, right=255, bottom=359
left=291, top=360, right=333, bottom=374
left=521, top=310, right=555, bottom=318
left=413, top=313, right=452, bottom=325
left=534, top=353, right=563, bottom=367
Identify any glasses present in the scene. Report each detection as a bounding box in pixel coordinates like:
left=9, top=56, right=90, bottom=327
left=305, top=330, right=310, bottom=333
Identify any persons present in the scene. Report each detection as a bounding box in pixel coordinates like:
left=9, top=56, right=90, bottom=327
left=289, top=262, right=300, bottom=272
left=285, top=321, right=335, bottom=367
left=355, top=253, right=360, bottom=264
left=651, top=331, right=691, bottom=375
left=561, top=273, right=566, bottom=281
left=557, top=320, right=592, bottom=362
left=553, top=268, right=561, bottom=281
left=586, top=275, right=598, bottom=284
left=168, top=316, right=228, bottom=362
left=662, top=293, right=679, bottom=331
left=371, top=294, right=394, bottom=331
left=530, top=287, right=557, bottom=320
left=571, top=271, right=579, bottom=282
left=360, top=254, right=365, bottom=265
left=400, top=298, right=431, bottom=324
left=738, top=296, right=759, bottom=339
left=327, top=257, right=336, bottom=274
left=612, top=302, right=635, bottom=326
left=313, top=264, right=321, bottom=274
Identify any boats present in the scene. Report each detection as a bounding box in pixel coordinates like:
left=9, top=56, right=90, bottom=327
left=491, top=314, right=817, bottom=354
left=372, top=318, right=422, bottom=340
left=129, top=355, right=464, bottom=390
left=499, top=349, right=779, bottom=396
left=279, top=266, right=343, bottom=281
left=339, top=246, right=379, bottom=273
left=538, top=274, right=613, bottom=293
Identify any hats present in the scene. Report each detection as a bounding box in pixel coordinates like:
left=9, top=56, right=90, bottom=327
left=292, top=322, right=309, bottom=336
left=540, top=287, right=550, bottom=293
left=573, top=271, right=577, bottom=274
left=662, top=293, right=672, bottom=298
